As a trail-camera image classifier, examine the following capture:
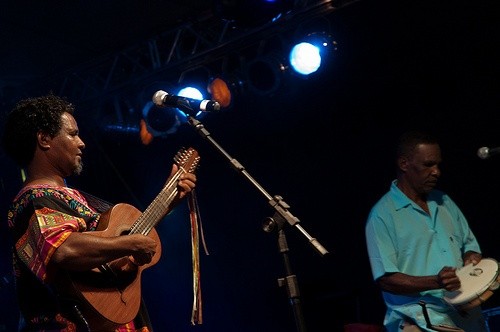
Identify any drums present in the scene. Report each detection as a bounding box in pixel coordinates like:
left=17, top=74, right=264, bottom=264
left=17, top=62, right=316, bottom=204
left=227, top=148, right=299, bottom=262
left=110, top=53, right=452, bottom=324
left=443, top=259, right=498, bottom=309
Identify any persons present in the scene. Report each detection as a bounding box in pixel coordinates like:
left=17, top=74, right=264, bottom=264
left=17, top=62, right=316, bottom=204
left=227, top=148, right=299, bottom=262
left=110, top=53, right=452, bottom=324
left=365, top=137, right=482, bottom=332
left=0, top=97, right=197, bottom=332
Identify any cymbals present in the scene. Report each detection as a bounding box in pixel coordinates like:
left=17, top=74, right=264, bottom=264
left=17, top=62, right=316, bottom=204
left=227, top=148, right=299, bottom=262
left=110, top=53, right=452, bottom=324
left=490, top=282, right=500, bottom=291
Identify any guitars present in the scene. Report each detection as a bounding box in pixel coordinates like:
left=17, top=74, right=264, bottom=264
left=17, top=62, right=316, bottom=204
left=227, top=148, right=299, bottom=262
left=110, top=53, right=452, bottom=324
left=68, top=146, right=201, bottom=330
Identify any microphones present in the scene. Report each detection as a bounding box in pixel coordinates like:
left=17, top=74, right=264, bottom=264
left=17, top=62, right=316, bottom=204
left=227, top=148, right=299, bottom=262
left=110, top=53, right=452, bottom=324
left=153, top=89, right=220, bottom=111
